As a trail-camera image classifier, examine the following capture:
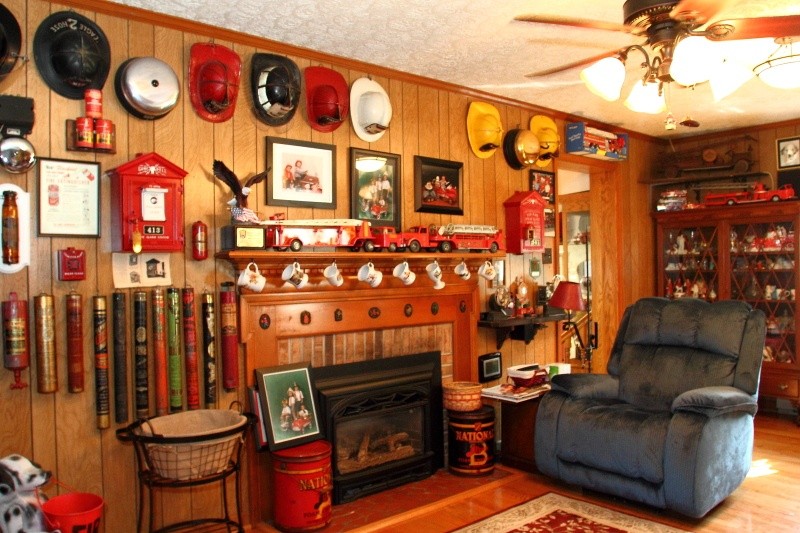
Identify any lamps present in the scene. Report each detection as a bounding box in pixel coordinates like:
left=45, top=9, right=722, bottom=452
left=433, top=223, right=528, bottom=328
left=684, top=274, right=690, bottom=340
left=579, top=8, right=800, bottom=130
left=503, top=128, right=541, bottom=170
left=550, top=280, right=598, bottom=373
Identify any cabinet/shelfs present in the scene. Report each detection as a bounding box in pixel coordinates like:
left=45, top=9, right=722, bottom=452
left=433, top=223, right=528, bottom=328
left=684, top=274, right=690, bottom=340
left=653, top=200, right=800, bottom=416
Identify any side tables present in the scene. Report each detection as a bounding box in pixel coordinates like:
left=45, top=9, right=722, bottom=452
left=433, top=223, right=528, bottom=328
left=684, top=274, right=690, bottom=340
left=500, top=397, right=541, bottom=474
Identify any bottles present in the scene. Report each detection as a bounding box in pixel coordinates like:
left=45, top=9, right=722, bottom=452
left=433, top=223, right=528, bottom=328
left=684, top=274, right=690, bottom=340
left=2, top=191, right=20, bottom=263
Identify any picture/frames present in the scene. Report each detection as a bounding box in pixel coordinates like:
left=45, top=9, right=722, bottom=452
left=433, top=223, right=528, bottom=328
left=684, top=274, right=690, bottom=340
left=414, top=155, right=464, bottom=216
left=529, top=169, right=555, bottom=205
left=249, top=360, right=325, bottom=451
left=776, top=137, right=800, bottom=171
left=36, top=156, right=103, bottom=240
left=349, top=147, right=402, bottom=233
left=265, top=136, right=337, bottom=209
left=477, top=351, right=502, bottom=384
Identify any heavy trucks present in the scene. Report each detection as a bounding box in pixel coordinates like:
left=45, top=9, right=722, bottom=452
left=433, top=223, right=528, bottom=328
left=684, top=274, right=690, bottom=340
left=400, top=223, right=507, bottom=253
left=259, top=218, right=407, bottom=252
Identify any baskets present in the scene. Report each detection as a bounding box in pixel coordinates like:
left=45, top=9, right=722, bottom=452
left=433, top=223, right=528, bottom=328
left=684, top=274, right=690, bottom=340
left=442, top=381, right=482, bottom=412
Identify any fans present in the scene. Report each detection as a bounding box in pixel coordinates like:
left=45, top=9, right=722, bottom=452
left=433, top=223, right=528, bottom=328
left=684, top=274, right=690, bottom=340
left=512, top=0, right=800, bottom=97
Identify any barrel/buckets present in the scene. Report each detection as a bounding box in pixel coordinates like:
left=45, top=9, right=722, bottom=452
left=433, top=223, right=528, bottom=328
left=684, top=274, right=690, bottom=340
left=35, top=478, right=106, bottom=533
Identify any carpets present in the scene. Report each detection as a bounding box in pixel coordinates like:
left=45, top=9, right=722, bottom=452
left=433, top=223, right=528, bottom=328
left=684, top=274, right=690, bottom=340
left=442, top=489, right=710, bottom=533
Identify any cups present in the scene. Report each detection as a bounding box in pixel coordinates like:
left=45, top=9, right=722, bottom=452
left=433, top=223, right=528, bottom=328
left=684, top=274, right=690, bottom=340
left=477, top=261, right=496, bottom=280
left=282, top=262, right=309, bottom=289
left=358, top=262, right=383, bottom=287
left=237, top=262, right=266, bottom=293
left=453, top=262, right=471, bottom=280
left=425, top=261, right=446, bottom=289
left=324, top=262, right=344, bottom=286
left=776, top=288, right=795, bottom=301
left=392, top=262, right=416, bottom=285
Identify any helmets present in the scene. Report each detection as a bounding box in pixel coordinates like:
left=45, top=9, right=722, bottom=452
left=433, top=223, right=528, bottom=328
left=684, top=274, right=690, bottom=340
left=0, top=2, right=22, bottom=83
left=305, top=65, right=350, bottom=132
left=32, top=11, right=111, bottom=100
left=250, top=52, right=302, bottom=127
left=529, top=115, right=561, bottom=168
left=350, top=77, right=393, bottom=142
left=189, top=42, right=242, bottom=122
left=466, top=101, right=504, bottom=159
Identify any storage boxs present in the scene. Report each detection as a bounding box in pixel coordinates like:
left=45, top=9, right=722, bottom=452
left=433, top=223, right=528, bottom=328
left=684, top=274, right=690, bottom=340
left=565, top=122, right=628, bottom=162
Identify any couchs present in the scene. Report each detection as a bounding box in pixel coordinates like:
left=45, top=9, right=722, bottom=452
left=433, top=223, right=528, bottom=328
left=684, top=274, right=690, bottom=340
left=534, top=297, right=767, bottom=519
